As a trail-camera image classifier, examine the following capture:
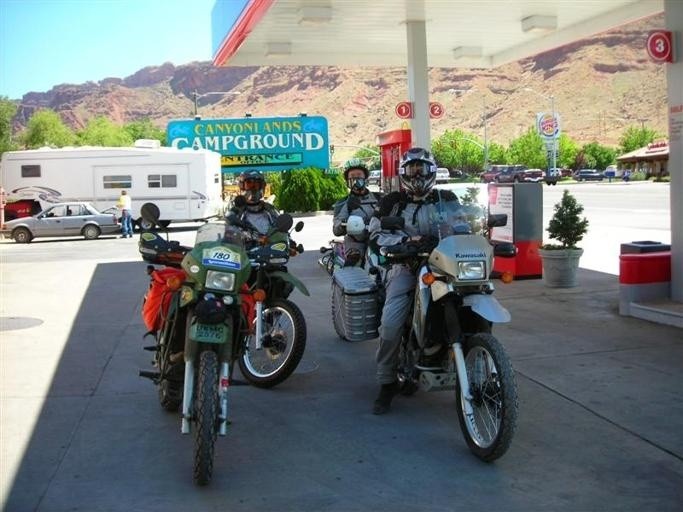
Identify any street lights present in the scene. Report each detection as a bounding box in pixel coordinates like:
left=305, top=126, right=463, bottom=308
left=193, top=87, right=241, bottom=117
left=520, top=85, right=557, bottom=177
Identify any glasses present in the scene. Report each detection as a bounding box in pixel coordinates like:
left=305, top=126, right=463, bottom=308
left=347, top=177, right=366, bottom=191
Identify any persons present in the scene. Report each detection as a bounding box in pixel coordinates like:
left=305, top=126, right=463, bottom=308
left=366, top=146, right=471, bottom=416
left=114, top=190, right=133, bottom=238
left=332, top=164, right=377, bottom=266
left=226, top=168, right=297, bottom=350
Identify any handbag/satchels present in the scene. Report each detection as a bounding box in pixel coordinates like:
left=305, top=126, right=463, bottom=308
left=142, top=266, right=266, bottom=337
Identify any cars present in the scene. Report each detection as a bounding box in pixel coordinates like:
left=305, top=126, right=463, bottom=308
left=1, top=201, right=120, bottom=243
left=362, top=164, right=601, bottom=187
left=3, top=187, right=72, bottom=221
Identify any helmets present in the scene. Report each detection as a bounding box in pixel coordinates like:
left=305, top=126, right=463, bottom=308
left=399, top=148, right=437, bottom=197
left=344, top=157, right=371, bottom=178
left=238, top=169, right=266, bottom=202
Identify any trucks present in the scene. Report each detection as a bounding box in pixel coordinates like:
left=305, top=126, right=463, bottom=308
left=1, top=139, right=223, bottom=231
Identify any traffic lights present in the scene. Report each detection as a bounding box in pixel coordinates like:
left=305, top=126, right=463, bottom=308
left=450, top=138, right=456, bottom=149
left=329, top=145, right=335, bottom=153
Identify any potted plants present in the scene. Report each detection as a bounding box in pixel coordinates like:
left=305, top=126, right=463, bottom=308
left=536, top=190, right=588, bottom=288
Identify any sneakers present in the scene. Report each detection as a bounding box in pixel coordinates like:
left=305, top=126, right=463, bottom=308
left=373, top=381, right=399, bottom=414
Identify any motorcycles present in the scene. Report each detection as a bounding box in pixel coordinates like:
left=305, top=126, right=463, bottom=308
left=222, top=196, right=314, bottom=389
left=315, top=196, right=390, bottom=283
left=136, top=202, right=292, bottom=488
left=332, top=180, right=521, bottom=462
